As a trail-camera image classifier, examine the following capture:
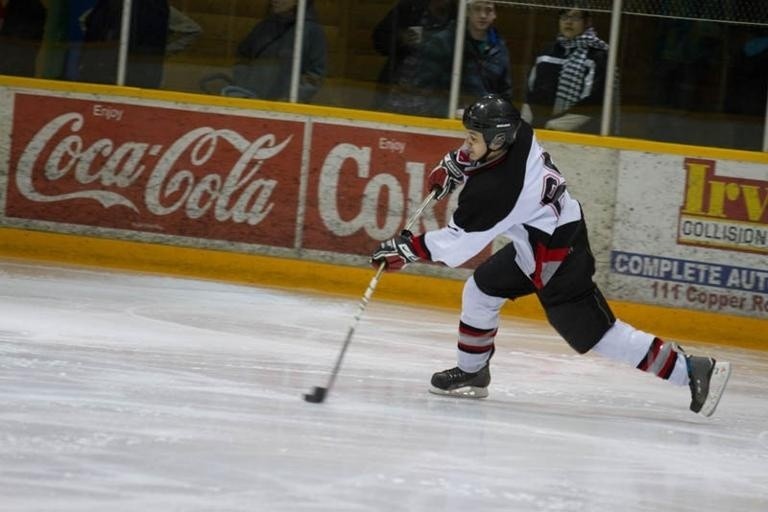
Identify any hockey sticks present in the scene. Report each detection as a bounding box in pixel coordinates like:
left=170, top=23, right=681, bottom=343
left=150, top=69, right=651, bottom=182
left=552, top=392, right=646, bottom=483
left=312, top=187, right=439, bottom=403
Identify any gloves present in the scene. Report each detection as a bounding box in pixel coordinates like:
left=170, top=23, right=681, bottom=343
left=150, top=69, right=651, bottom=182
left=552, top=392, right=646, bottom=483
left=427, top=148, right=478, bottom=201
left=368, top=235, right=421, bottom=272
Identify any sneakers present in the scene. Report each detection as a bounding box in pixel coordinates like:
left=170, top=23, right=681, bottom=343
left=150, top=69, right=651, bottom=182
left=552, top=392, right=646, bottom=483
left=684, top=354, right=716, bottom=413
left=431, top=366, right=490, bottom=390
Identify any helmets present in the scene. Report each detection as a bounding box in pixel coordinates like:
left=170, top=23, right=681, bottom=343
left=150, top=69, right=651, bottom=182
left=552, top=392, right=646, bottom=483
left=463, top=93, right=522, bottom=151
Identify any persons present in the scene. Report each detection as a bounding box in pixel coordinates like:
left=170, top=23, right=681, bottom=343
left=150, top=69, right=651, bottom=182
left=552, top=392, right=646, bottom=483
left=226, top=0, right=326, bottom=102
left=396, top=1, right=517, bottom=122
left=52, top=1, right=166, bottom=87
left=368, top=2, right=455, bottom=105
left=519, top=3, right=620, bottom=133
left=364, top=94, right=716, bottom=414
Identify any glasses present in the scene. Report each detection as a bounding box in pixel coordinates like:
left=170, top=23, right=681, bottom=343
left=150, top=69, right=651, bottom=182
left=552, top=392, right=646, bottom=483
left=559, top=14, right=584, bottom=21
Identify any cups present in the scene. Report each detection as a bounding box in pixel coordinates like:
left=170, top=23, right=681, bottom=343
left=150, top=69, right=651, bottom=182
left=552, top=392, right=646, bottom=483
left=409, top=25, right=424, bottom=44
